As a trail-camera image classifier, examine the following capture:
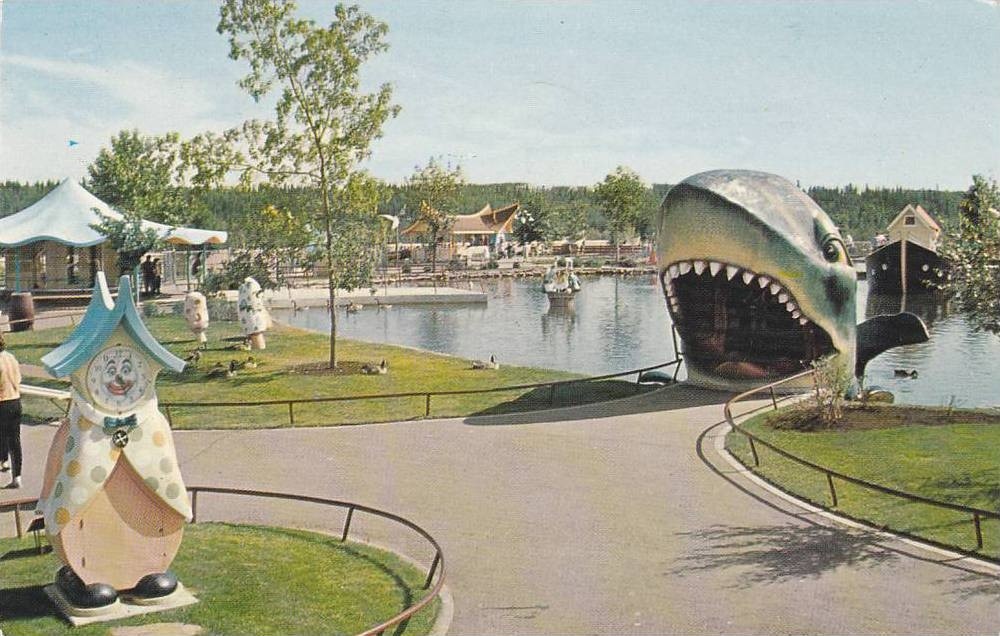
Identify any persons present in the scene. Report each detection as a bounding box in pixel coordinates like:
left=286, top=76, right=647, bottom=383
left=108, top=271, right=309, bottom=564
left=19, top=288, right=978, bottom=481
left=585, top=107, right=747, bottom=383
left=0, top=335, right=23, bottom=489
left=0, top=247, right=208, bottom=295
left=399, top=247, right=410, bottom=265
left=546, top=266, right=581, bottom=291
left=476, top=238, right=516, bottom=258
left=875, top=233, right=882, bottom=245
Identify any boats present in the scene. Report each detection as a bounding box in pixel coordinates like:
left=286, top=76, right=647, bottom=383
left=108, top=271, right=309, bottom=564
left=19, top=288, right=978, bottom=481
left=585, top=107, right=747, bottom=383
left=542, top=256, right=581, bottom=299
left=864, top=207, right=954, bottom=297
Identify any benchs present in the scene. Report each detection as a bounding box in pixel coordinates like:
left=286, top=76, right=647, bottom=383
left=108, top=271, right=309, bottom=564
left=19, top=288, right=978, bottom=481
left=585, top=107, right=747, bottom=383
left=33, top=295, right=93, bottom=307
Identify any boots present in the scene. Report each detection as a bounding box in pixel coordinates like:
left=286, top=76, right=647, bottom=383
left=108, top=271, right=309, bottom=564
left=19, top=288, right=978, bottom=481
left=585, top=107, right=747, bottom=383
left=0, top=462, right=10, bottom=471
left=5, top=476, right=21, bottom=488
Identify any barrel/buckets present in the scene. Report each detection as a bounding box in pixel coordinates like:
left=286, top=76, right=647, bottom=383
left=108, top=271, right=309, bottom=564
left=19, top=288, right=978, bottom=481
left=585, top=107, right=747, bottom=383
left=9, top=292, right=35, bottom=331
